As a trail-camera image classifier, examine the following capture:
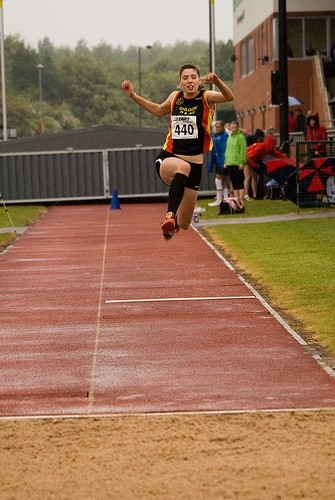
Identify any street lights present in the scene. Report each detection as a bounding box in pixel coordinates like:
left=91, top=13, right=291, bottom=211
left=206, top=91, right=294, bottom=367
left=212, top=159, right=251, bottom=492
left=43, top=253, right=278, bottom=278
left=37, top=63, right=45, bottom=135
left=136, top=45, right=152, bottom=126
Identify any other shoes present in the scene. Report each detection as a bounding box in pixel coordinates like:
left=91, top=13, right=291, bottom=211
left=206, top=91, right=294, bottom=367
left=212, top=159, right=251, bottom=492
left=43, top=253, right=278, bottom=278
left=244, top=195, right=251, bottom=201
left=208, top=201, right=221, bottom=207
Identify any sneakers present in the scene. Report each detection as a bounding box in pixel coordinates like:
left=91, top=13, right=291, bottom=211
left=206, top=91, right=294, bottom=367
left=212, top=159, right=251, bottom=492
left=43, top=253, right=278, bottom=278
left=160, top=211, right=176, bottom=240
left=174, top=214, right=179, bottom=233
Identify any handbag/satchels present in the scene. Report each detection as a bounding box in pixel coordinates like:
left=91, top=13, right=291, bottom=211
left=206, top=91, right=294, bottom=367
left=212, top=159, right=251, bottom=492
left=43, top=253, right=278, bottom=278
left=218, top=191, right=245, bottom=215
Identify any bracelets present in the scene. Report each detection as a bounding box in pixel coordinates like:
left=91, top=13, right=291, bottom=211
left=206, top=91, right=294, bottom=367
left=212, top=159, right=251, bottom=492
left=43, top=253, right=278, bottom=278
left=130, top=92, right=136, bottom=99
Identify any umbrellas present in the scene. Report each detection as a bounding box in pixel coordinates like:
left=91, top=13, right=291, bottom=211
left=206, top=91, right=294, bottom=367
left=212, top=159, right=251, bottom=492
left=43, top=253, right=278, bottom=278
left=267, top=157, right=335, bottom=210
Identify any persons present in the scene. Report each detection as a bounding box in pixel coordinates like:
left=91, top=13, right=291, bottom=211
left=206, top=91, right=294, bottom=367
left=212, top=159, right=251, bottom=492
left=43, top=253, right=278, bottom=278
left=122, top=64, right=235, bottom=241
left=288, top=108, right=307, bottom=142
left=305, top=111, right=328, bottom=160
left=208, top=122, right=283, bottom=207
